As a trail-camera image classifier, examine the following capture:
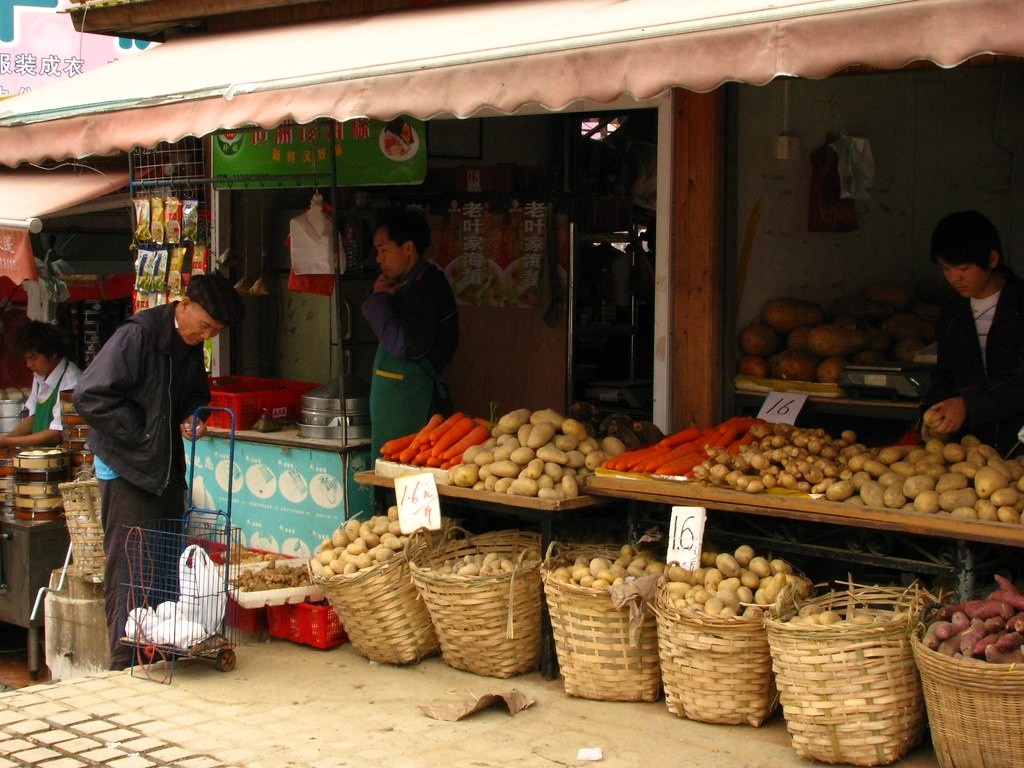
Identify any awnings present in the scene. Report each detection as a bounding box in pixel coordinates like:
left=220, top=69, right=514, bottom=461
left=1, top=0, right=1024, bottom=168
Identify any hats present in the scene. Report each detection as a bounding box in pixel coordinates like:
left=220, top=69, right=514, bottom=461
left=186, top=275, right=247, bottom=326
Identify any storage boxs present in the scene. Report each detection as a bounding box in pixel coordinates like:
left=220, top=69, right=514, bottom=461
left=268, top=603, right=351, bottom=652
left=220, top=558, right=325, bottom=609
left=205, top=375, right=324, bottom=431
left=735, top=375, right=847, bottom=399
left=187, top=548, right=300, bottom=634
left=186, top=537, right=228, bottom=557
left=450, top=163, right=514, bottom=195
left=374, top=457, right=448, bottom=485
left=509, top=165, right=535, bottom=192
left=571, top=195, right=635, bottom=234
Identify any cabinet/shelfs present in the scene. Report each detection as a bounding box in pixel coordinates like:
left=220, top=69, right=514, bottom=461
left=575, top=231, right=639, bottom=380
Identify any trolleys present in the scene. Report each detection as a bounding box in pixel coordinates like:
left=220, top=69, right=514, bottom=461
left=116, top=407, right=236, bottom=672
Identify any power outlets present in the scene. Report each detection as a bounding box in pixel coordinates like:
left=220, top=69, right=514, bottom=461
left=775, top=135, right=802, bottom=162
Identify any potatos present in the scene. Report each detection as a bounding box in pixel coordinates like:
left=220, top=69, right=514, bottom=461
left=923, top=573, right=1024, bottom=665
left=443, top=403, right=663, bottom=499
left=825, top=409, right=1024, bottom=525
left=426, top=551, right=517, bottom=576
left=310, top=505, right=418, bottom=577
left=554, top=543, right=664, bottom=589
left=659, top=544, right=875, bottom=627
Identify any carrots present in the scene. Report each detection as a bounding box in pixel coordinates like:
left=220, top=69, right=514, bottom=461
left=601, top=417, right=770, bottom=479
left=379, top=411, right=493, bottom=468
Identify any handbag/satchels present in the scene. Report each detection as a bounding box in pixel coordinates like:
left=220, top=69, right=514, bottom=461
left=179, top=544, right=229, bottom=636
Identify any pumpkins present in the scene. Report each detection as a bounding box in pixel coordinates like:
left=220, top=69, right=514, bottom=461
left=740, top=278, right=947, bottom=384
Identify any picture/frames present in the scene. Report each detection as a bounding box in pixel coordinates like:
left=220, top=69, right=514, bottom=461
left=425, top=118, right=484, bottom=160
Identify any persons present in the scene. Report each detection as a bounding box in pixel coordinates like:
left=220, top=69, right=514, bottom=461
left=919, top=209, right=1024, bottom=461
left=70, top=275, right=245, bottom=673
left=1, top=320, right=81, bottom=449
left=361, top=205, right=459, bottom=513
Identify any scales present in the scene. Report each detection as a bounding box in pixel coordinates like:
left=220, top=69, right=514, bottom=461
left=838, top=363, right=930, bottom=401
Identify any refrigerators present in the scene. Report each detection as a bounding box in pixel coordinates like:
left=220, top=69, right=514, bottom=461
left=275, top=274, right=379, bottom=399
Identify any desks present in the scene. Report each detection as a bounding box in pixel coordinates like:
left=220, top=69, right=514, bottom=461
left=353, top=470, right=608, bottom=682
left=584, top=473, right=1024, bottom=608
left=182, top=425, right=374, bottom=559
left=735, top=390, right=925, bottom=426
